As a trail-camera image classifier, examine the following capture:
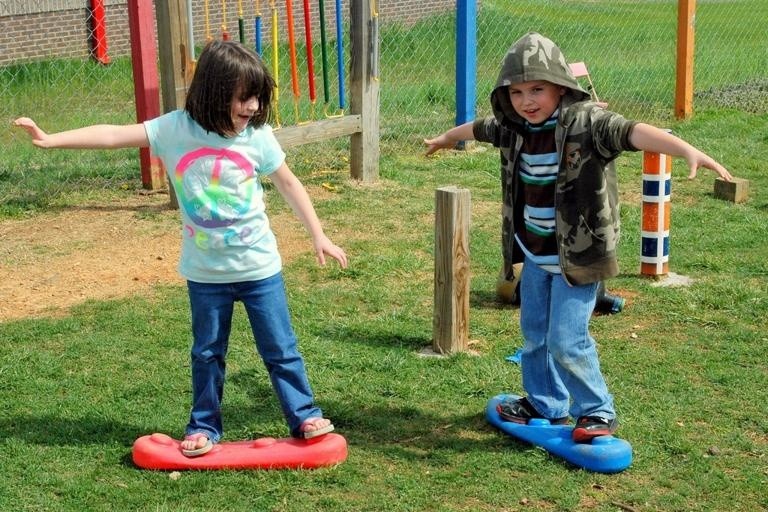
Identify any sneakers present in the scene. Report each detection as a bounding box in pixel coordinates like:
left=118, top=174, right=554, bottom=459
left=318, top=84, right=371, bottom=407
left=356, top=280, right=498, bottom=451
left=573, top=416, right=619, bottom=441
left=497, top=395, right=568, bottom=426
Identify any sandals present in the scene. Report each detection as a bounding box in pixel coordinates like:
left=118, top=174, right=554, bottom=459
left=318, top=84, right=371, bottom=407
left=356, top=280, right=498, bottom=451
left=181, top=434, right=212, bottom=456
left=301, top=417, right=336, bottom=439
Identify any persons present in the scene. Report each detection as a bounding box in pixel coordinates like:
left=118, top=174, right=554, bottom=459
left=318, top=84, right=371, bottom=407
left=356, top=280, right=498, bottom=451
left=423, top=30, right=733, bottom=441
left=10, top=40, right=347, bottom=458
